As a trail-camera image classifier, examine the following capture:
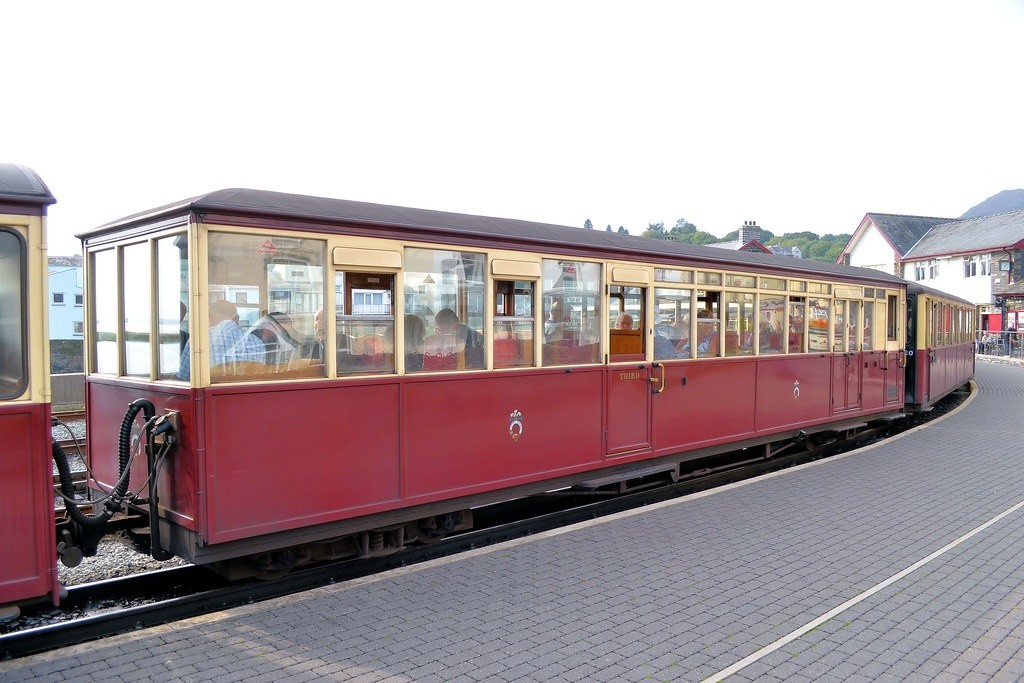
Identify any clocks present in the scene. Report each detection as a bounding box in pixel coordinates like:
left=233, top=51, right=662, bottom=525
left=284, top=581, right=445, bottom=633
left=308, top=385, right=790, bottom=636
left=999, top=260, right=1012, bottom=271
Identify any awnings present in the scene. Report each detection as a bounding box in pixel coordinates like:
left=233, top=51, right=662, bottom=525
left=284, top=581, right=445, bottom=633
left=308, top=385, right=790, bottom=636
left=914, top=276, right=991, bottom=305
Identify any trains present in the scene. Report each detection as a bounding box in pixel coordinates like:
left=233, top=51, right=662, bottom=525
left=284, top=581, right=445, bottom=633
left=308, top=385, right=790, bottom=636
left=1, top=163, right=974, bottom=615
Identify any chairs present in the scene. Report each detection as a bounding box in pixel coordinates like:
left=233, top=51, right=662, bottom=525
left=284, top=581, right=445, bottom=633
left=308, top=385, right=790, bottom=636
left=976, top=339, right=1006, bottom=356
left=352, top=324, right=873, bottom=375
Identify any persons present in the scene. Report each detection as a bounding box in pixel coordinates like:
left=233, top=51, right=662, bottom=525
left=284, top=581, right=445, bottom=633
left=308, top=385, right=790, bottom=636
left=132, top=299, right=485, bottom=382
left=545, top=305, right=803, bottom=361
left=982, top=323, right=1018, bottom=358
